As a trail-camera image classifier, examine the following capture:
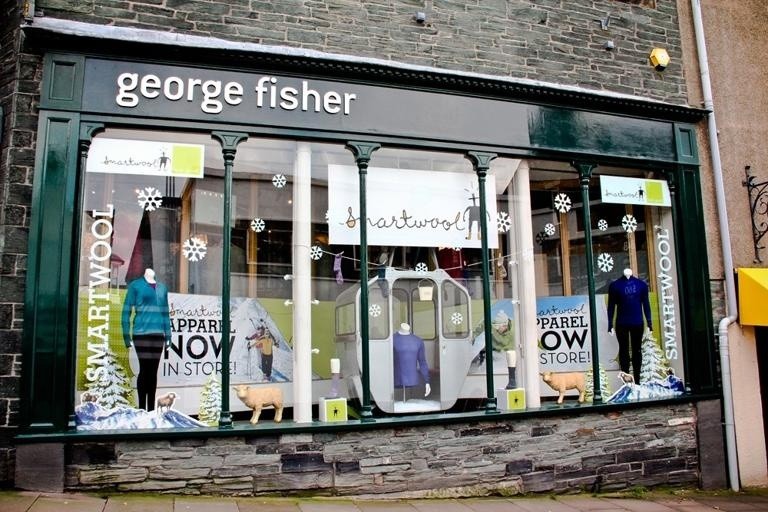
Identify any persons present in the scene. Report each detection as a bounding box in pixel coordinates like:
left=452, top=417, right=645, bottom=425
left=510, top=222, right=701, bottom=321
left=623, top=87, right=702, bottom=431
left=245, top=325, right=280, bottom=381
left=607, top=268, right=654, bottom=385
left=121, top=268, right=173, bottom=412
left=503, top=350, right=517, bottom=388
left=393, top=323, right=432, bottom=397
left=467, top=309, right=515, bottom=367
